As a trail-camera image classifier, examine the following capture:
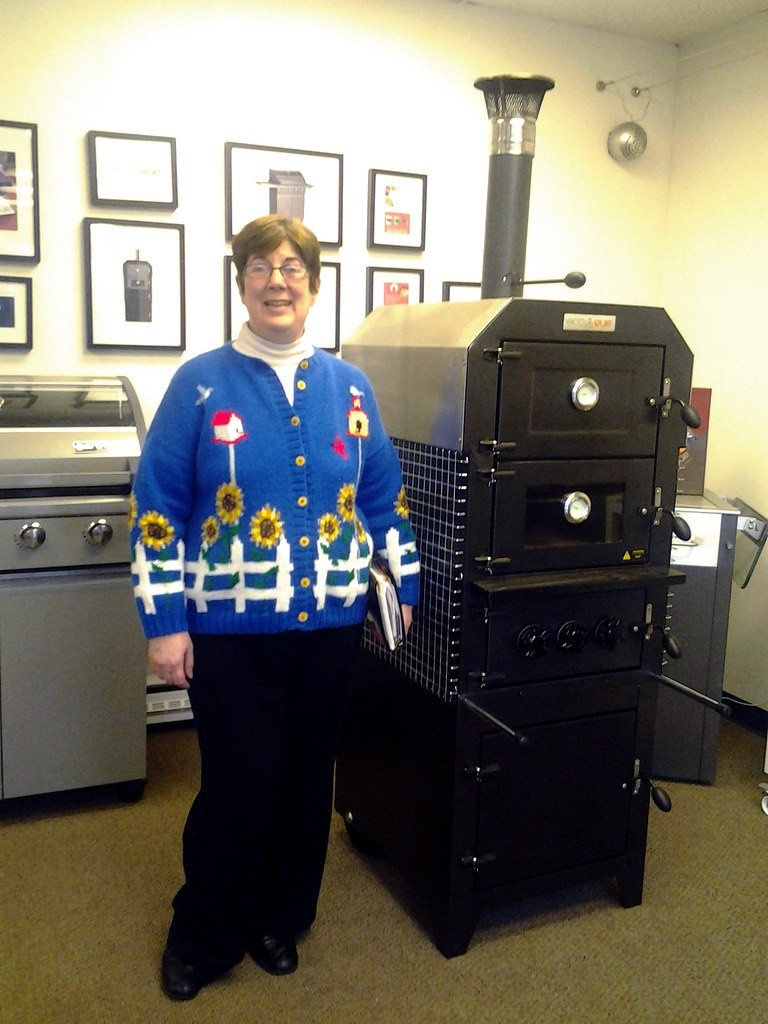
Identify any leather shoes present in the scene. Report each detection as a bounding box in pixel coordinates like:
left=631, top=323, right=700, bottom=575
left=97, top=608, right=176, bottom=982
left=159, top=960, right=199, bottom=1001
left=248, top=936, right=298, bottom=976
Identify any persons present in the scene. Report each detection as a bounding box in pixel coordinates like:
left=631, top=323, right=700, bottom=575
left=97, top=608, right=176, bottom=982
left=131, top=215, right=420, bottom=1002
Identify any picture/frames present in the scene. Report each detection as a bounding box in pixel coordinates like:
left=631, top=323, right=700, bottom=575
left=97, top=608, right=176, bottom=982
left=225, top=141, right=344, bottom=249
left=223, top=254, right=341, bottom=353
left=0, top=120, right=42, bottom=265
left=366, top=168, right=428, bottom=253
left=82, top=217, right=189, bottom=352
left=441, top=281, right=483, bottom=302
left=364, top=266, right=425, bottom=318
left=0, top=274, right=34, bottom=352
left=88, top=128, right=180, bottom=212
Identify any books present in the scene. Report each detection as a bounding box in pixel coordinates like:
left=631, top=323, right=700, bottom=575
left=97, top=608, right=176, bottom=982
left=370, top=555, right=407, bottom=652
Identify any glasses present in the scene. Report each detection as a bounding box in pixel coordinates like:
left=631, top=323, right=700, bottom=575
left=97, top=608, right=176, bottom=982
left=244, top=263, right=310, bottom=280
